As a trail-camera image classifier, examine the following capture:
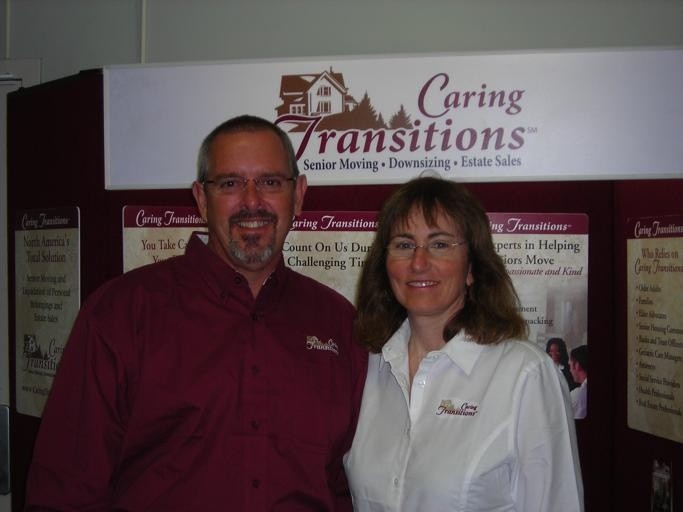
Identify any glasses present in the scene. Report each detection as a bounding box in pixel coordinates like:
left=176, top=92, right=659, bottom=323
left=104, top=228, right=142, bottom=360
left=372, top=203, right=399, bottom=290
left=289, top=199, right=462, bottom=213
left=382, top=235, right=467, bottom=257
left=200, top=175, right=296, bottom=192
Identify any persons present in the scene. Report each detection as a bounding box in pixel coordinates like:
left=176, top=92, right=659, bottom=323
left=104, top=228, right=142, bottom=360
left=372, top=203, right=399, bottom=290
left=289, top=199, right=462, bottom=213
left=24, top=113, right=368, bottom=512
left=341, top=167, right=587, bottom=512
left=567, top=345, right=588, bottom=420
left=545, top=337, right=580, bottom=392
